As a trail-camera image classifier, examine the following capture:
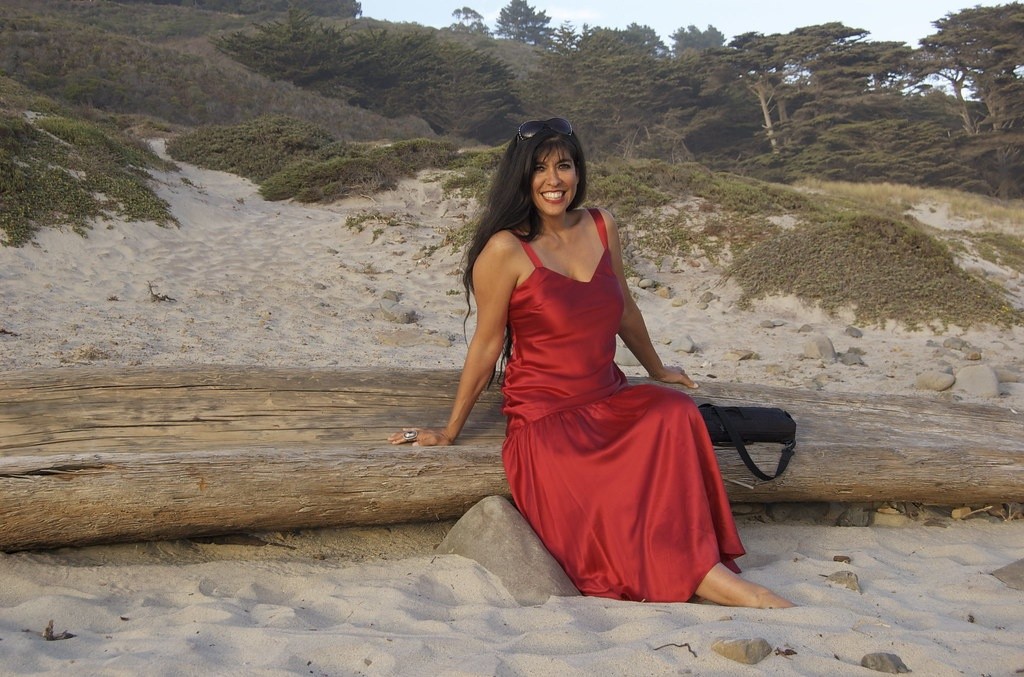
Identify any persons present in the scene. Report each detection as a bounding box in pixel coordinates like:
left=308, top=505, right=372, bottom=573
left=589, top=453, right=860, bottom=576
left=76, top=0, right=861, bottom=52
left=387, top=117, right=797, bottom=609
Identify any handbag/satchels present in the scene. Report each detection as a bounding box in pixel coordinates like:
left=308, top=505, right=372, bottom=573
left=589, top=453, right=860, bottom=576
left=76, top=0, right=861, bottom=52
left=697, top=402, right=799, bottom=480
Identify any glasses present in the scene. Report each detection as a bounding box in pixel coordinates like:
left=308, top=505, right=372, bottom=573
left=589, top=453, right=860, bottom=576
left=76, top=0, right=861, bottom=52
left=517, top=118, right=574, bottom=141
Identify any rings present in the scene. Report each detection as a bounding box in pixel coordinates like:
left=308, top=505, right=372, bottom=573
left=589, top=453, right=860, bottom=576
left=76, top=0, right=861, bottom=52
left=403, top=430, right=417, bottom=441
